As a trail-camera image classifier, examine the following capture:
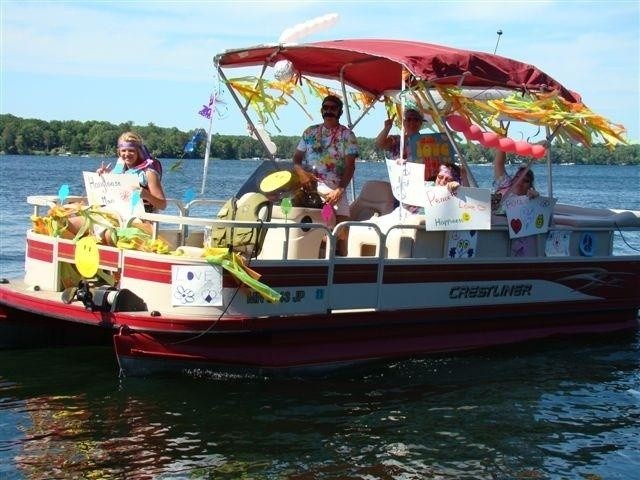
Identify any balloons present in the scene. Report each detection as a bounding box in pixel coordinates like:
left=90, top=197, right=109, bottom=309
left=446, top=114, right=546, bottom=158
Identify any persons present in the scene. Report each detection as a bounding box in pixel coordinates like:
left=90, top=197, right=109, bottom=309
left=95, top=130, right=166, bottom=216
left=432, top=159, right=462, bottom=199
left=492, top=118, right=543, bottom=210
left=289, top=91, right=361, bottom=207
left=375, top=104, right=429, bottom=166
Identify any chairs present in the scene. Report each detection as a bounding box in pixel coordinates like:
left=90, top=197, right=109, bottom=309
left=177, top=192, right=269, bottom=258
left=348, top=180, right=394, bottom=221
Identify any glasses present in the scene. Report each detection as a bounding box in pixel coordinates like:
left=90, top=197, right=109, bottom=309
left=437, top=174, right=453, bottom=182
left=323, top=105, right=337, bottom=112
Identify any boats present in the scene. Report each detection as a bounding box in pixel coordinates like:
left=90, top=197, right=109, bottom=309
left=0, top=39, right=640, bottom=379
